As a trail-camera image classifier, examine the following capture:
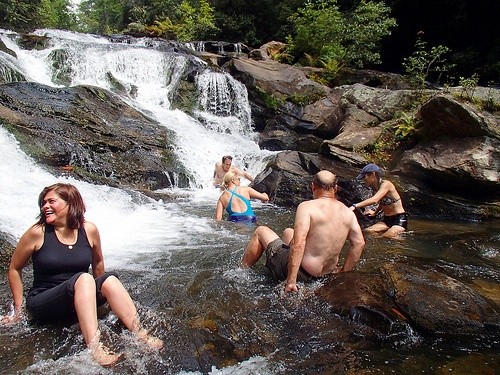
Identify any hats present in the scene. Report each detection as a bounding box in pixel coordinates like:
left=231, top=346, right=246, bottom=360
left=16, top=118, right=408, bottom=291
left=355, top=163, right=381, bottom=180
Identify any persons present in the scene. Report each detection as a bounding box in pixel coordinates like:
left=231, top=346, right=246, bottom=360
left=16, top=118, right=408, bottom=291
left=1, top=182, right=164, bottom=368
left=236, top=169, right=366, bottom=298
left=212, top=156, right=255, bottom=192
left=348, top=162, right=409, bottom=242
left=215, top=170, right=268, bottom=226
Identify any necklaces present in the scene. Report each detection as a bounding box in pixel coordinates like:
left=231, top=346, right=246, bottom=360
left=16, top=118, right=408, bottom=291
left=56, top=227, right=78, bottom=250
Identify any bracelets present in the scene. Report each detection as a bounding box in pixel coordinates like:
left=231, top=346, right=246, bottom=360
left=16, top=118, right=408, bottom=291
left=353, top=203, right=358, bottom=209
